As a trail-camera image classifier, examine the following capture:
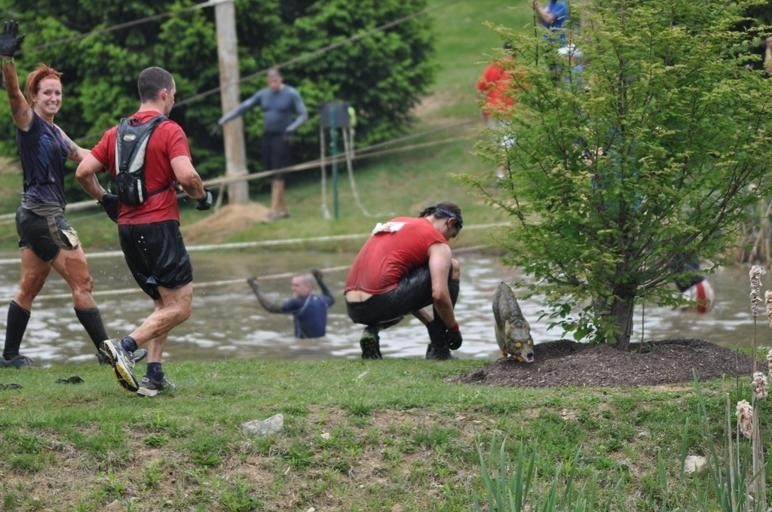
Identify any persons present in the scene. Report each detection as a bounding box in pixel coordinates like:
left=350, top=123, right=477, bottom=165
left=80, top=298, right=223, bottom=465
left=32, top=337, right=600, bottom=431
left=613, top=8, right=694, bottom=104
left=248, top=269, right=335, bottom=338
left=475, top=0, right=587, bottom=183
left=75, top=66, right=213, bottom=396
left=0, top=19, right=147, bottom=367
left=207, top=67, right=308, bottom=221
left=344, top=202, right=464, bottom=359
left=671, top=246, right=714, bottom=315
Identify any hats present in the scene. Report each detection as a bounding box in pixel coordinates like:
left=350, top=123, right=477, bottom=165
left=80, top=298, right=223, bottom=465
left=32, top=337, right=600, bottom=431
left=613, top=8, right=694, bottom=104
left=557, top=43, right=582, bottom=57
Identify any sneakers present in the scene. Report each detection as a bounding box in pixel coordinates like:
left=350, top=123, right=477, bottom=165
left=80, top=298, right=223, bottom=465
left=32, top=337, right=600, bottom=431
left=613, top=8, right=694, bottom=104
left=137, top=374, right=177, bottom=395
left=98, top=337, right=147, bottom=392
left=426, top=343, right=455, bottom=361
left=0, top=355, right=40, bottom=369
left=260, top=211, right=288, bottom=224
left=360, top=327, right=382, bottom=361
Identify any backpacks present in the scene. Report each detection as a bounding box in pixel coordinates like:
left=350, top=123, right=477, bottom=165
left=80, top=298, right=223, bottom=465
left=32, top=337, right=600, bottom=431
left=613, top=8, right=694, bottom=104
left=116, top=114, right=169, bottom=205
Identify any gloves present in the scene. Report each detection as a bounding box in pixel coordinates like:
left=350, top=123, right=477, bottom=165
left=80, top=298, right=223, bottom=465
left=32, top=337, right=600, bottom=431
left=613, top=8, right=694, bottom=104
left=428, top=324, right=462, bottom=350
left=196, top=188, right=213, bottom=210
left=101, top=194, right=118, bottom=223
